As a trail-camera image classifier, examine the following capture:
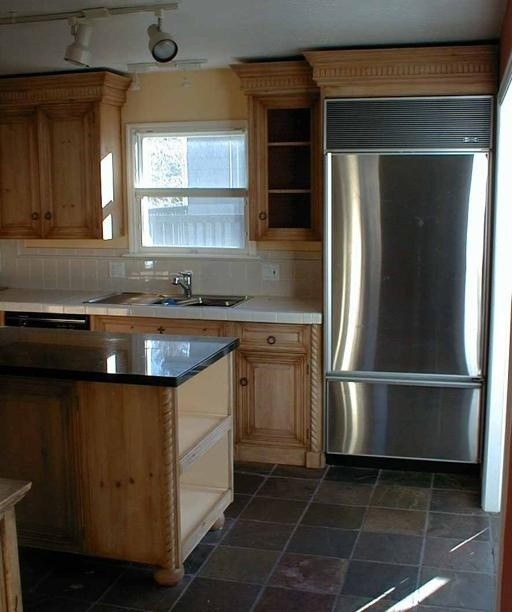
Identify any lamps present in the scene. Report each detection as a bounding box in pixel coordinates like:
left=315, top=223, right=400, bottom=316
left=147, top=9, right=179, bottom=66
left=64, top=14, right=92, bottom=71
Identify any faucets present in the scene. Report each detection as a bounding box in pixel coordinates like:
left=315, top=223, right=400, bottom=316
left=169, top=268, right=193, bottom=299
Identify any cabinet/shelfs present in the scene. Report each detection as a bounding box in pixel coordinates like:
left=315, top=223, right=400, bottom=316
left=0, top=71, right=133, bottom=242
left=229, top=61, right=323, bottom=242
left=230, top=321, right=324, bottom=470
left=89, top=316, right=229, bottom=339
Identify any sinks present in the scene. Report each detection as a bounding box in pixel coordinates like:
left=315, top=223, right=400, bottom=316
left=176, top=293, right=255, bottom=309
left=85, top=289, right=146, bottom=306
left=152, top=295, right=184, bottom=307
